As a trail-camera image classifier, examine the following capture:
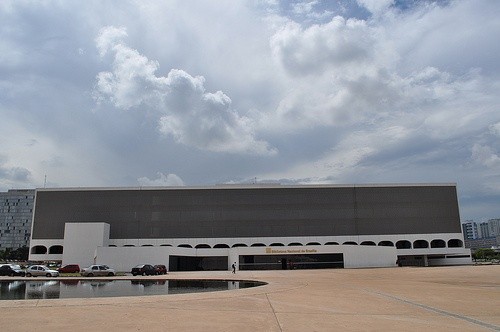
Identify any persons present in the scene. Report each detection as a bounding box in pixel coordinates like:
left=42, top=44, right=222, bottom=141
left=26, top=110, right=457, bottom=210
left=232, top=261, right=236, bottom=274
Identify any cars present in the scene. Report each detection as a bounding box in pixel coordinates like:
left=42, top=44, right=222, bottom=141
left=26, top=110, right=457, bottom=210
left=56, top=264, right=80, bottom=273
left=81, top=264, right=117, bottom=277
left=153, top=264, right=167, bottom=274
left=20, top=262, right=62, bottom=269
left=0, top=264, right=25, bottom=277
left=131, top=263, right=161, bottom=276
left=25, top=264, right=60, bottom=277
